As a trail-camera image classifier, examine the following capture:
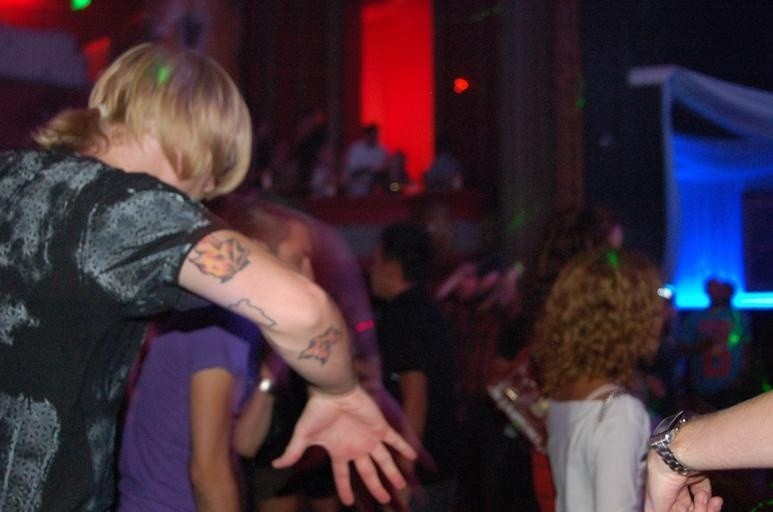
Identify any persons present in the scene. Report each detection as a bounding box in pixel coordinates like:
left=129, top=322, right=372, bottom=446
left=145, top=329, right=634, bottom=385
left=116, top=189, right=316, bottom=510
left=205, top=108, right=772, bottom=512
left=0, top=36, right=421, bottom=511
